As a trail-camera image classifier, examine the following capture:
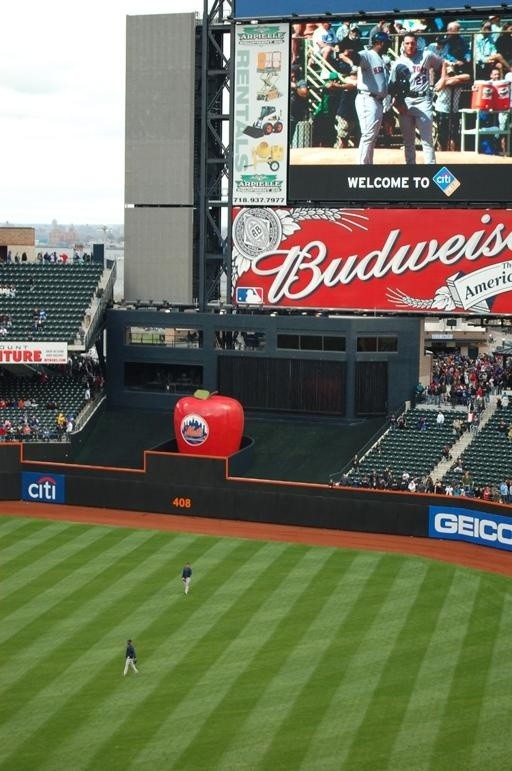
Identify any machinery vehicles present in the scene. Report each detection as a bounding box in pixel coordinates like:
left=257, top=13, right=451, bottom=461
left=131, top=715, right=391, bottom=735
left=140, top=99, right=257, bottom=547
left=240, top=104, right=285, bottom=137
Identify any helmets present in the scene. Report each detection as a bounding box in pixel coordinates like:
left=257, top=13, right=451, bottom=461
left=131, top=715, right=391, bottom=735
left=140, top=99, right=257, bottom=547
left=371, top=32, right=393, bottom=42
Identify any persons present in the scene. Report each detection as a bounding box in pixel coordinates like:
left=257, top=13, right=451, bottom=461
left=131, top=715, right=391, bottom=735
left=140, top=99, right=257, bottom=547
left=0, top=246, right=107, bottom=443
left=122, top=640, right=139, bottom=676
left=328, top=342, right=512, bottom=508
left=289, top=16, right=512, bottom=158
left=180, top=562, right=193, bottom=596
left=341, top=31, right=390, bottom=164
left=387, top=31, right=446, bottom=165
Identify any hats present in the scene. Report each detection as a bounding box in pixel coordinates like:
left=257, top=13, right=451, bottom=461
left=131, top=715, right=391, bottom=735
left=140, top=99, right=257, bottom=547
left=447, top=66, right=456, bottom=76
left=349, top=23, right=358, bottom=31
left=322, top=73, right=338, bottom=80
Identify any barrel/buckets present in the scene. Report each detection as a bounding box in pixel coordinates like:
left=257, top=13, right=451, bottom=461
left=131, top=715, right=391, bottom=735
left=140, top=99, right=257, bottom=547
left=492, top=80, right=511, bottom=111
left=470, top=79, right=497, bottom=113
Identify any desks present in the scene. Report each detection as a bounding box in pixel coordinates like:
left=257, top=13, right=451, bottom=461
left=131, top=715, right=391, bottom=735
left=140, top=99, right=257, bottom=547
left=458, top=109, right=512, bottom=155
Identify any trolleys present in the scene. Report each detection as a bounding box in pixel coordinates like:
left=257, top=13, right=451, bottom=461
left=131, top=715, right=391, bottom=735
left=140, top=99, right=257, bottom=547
left=240, top=142, right=284, bottom=172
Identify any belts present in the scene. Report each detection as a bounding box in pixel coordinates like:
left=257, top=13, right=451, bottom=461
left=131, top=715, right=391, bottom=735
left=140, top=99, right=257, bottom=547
left=402, top=92, right=426, bottom=97
left=358, top=90, right=384, bottom=101
